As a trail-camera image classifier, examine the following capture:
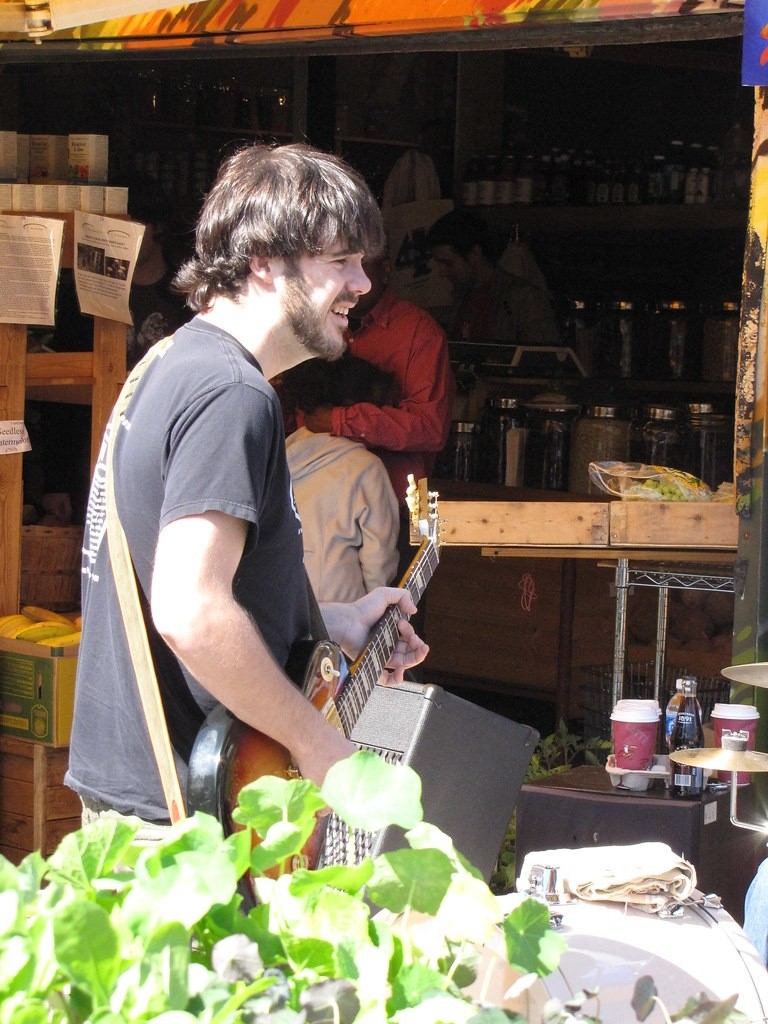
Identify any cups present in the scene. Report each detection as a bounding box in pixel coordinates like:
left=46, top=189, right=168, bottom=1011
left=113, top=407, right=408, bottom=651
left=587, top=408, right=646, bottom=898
left=616, top=699, right=663, bottom=717
left=610, top=704, right=660, bottom=772
left=709, top=703, right=760, bottom=785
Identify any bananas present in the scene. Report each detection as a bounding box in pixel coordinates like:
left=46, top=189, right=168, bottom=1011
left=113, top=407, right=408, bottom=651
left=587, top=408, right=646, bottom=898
left=0, top=606, right=82, bottom=648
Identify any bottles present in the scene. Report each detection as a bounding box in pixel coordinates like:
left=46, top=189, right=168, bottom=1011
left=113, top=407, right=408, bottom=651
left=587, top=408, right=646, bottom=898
left=462, top=141, right=721, bottom=207
left=664, top=679, right=702, bottom=788
left=668, top=676, right=704, bottom=799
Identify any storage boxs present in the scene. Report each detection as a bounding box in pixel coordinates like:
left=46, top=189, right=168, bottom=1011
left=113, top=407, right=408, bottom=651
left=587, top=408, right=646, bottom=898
left=610, top=501, right=739, bottom=553
left=0, top=629, right=79, bottom=749
left=0, top=130, right=128, bottom=216
left=408, top=502, right=609, bottom=550
left=21, top=524, right=87, bottom=613
left=0, top=739, right=82, bottom=866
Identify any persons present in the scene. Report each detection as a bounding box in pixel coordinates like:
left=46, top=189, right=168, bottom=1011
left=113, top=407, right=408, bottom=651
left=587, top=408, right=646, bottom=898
left=63, top=142, right=430, bottom=915
left=278, top=239, right=452, bottom=684
left=21, top=171, right=195, bottom=527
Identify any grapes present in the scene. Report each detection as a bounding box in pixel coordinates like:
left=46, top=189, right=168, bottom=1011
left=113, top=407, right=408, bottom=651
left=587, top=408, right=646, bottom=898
left=621, top=480, right=712, bottom=502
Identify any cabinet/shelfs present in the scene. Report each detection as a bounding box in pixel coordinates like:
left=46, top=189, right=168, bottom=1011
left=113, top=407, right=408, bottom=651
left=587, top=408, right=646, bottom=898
left=516, top=767, right=756, bottom=917
left=1, top=214, right=148, bottom=620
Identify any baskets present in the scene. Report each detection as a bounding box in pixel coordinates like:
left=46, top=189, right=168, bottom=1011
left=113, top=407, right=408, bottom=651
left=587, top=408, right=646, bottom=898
left=579, top=659, right=731, bottom=760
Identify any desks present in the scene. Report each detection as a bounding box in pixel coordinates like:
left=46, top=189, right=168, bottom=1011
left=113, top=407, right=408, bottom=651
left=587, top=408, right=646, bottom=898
left=481, top=546, right=739, bottom=766
left=415, top=892, right=768, bottom=1024
